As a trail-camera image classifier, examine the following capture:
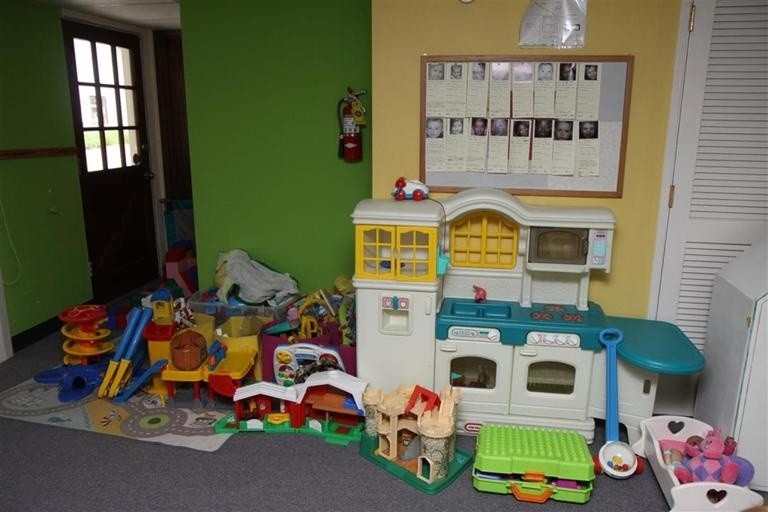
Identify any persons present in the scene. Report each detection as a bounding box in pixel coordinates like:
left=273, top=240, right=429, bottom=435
left=428, top=63, right=597, bottom=80
left=426, top=116, right=598, bottom=140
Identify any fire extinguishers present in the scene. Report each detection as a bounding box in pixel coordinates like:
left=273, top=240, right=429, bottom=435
left=338, top=87, right=366, bottom=161
